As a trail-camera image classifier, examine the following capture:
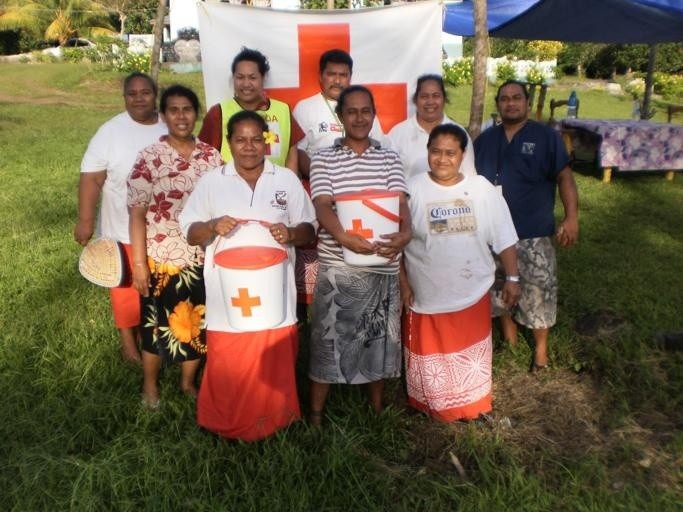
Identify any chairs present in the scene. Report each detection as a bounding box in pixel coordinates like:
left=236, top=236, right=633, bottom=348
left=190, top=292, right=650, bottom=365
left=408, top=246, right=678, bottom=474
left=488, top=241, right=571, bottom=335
left=547, top=96, right=581, bottom=121
left=488, top=80, right=550, bottom=129
left=668, top=103, right=683, bottom=120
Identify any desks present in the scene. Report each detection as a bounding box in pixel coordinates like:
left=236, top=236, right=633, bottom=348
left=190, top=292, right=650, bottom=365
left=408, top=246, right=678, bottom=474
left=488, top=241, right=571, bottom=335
left=559, top=115, right=682, bottom=185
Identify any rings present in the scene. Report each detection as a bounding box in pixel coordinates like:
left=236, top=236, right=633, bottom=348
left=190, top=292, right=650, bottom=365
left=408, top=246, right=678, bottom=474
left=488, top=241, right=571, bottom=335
left=279, top=234, right=283, bottom=240
left=276, top=228, right=281, bottom=234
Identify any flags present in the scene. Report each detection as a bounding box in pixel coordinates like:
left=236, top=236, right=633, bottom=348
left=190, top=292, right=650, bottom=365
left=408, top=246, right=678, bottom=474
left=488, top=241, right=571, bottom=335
left=194, top=0, right=448, bottom=130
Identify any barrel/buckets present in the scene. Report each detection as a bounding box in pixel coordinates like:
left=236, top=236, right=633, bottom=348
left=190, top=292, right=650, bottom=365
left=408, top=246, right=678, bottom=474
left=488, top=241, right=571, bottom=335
left=210, top=218, right=289, bottom=331
left=333, top=189, right=401, bottom=267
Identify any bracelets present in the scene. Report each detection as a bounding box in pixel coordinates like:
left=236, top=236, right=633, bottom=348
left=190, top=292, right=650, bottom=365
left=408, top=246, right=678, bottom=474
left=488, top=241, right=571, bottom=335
left=504, top=275, right=522, bottom=283
left=132, top=261, right=148, bottom=266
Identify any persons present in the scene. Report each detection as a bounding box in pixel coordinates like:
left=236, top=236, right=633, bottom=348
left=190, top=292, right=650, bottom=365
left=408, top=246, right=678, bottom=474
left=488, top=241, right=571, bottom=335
left=291, top=47, right=385, bottom=332
left=469, top=75, right=579, bottom=384
left=72, top=68, right=173, bottom=367
left=192, top=46, right=307, bottom=175
left=178, top=108, right=321, bottom=445
left=124, top=81, right=225, bottom=412
left=397, top=120, right=521, bottom=422
left=305, top=83, right=412, bottom=430
left=386, top=71, right=480, bottom=190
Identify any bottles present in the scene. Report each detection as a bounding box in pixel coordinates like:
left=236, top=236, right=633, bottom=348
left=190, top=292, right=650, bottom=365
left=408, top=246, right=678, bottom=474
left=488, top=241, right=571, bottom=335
left=567, top=90, right=577, bottom=119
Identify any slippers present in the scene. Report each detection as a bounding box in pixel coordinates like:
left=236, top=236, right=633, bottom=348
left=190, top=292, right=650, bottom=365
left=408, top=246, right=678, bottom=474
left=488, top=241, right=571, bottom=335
left=533, top=352, right=551, bottom=372
left=141, top=399, right=160, bottom=410
left=493, top=342, right=517, bottom=354
